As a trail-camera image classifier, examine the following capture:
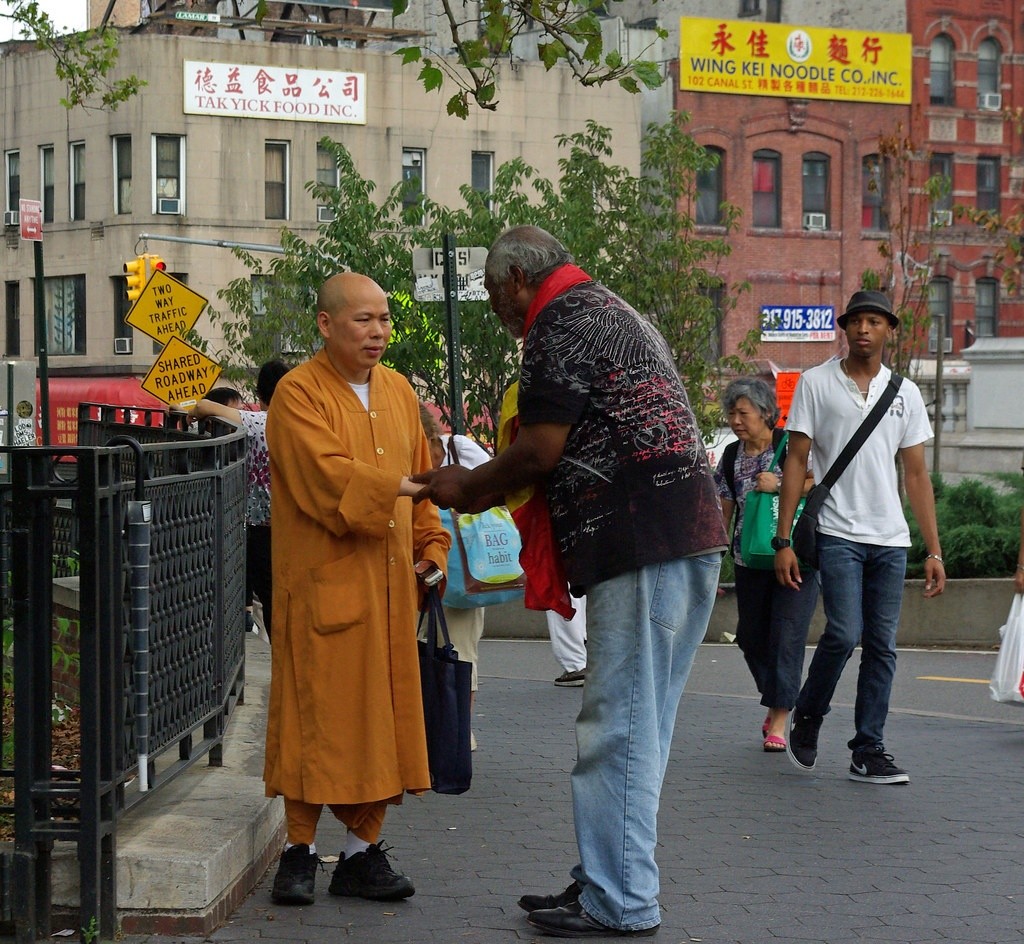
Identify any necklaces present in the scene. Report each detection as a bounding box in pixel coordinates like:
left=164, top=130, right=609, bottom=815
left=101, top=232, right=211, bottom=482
left=843, top=358, right=871, bottom=394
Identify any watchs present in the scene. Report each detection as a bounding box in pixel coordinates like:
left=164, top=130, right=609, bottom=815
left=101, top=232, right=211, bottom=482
left=770, top=536, right=790, bottom=551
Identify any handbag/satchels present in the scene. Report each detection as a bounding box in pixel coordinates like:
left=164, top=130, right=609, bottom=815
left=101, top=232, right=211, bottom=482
left=792, top=483, right=830, bottom=570
left=437, top=434, right=529, bottom=607
left=989, top=592, right=1024, bottom=706
left=742, top=433, right=818, bottom=574
left=417, top=583, right=472, bottom=795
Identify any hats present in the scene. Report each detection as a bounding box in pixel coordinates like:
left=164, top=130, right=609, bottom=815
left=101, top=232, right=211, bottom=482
left=837, top=290, right=899, bottom=330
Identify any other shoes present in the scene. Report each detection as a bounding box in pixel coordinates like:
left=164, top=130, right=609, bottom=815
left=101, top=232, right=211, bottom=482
left=554, top=667, right=587, bottom=687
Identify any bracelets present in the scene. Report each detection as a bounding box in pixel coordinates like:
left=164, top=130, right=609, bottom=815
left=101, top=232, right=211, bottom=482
left=925, top=553, right=945, bottom=566
left=1017, top=564, right=1024, bottom=571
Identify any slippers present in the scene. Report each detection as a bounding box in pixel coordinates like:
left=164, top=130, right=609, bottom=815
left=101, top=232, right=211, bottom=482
left=762, top=716, right=788, bottom=752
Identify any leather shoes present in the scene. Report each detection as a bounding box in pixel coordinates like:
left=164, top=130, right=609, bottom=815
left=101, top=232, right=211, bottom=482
left=518, top=882, right=661, bottom=938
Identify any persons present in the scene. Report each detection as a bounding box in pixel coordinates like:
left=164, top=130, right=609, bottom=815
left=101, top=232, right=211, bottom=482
left=711, top=377, right=820, bottom=753
left=262, top=274, right=452, bottom=907
left=770, top=293, right=945, bottom=786
left=1013, top=453, right=1024, bottom=596
left=416, top=396, right=494, bottom=749
left=497, top=379, right=589, bottom=688
left=163, top=358, right=291, bottom=645
left=408, top=224, right=731, bottom=937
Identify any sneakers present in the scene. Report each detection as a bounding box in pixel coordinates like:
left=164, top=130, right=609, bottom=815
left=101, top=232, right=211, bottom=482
left=328, top=840, right=415, bottom=901
left=271, top=842, right=324, bottom=906
left=786, top=707, right=822, bottom=772
left=849, top=748, right=909, bottom=784
